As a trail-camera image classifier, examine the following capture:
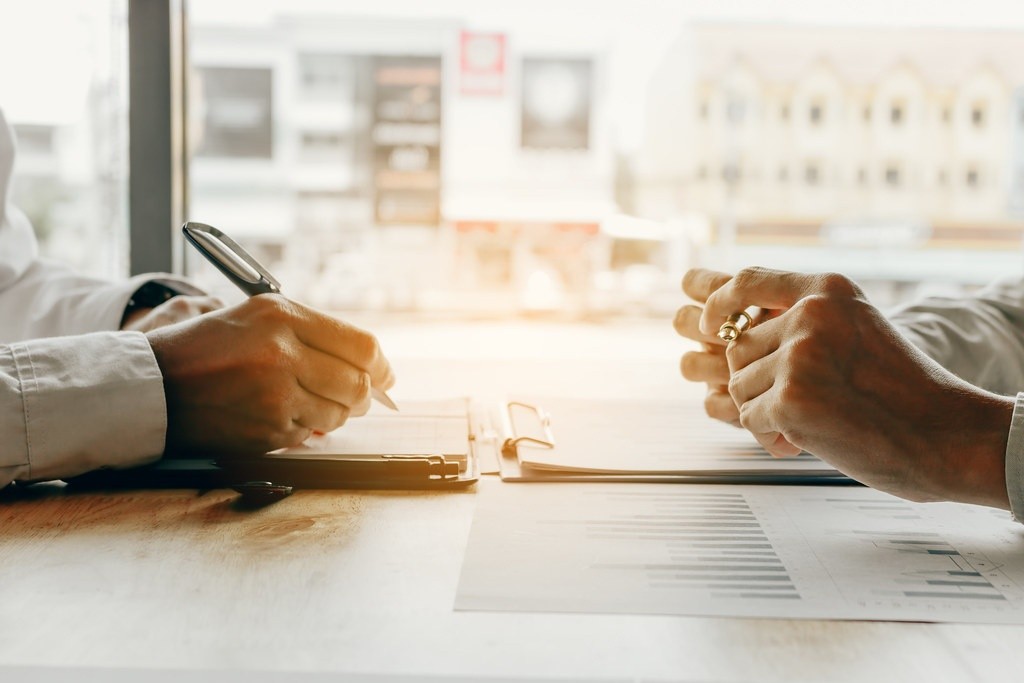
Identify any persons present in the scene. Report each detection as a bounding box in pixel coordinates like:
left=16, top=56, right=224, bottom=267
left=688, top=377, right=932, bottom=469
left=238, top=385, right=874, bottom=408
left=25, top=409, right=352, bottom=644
left=0, top=107, right=397, bottom=487
left=672, top=265, right=1016, bottom=516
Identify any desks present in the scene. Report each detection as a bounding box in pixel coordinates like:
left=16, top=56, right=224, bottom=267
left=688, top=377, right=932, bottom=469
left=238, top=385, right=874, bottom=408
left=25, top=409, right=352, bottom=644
left=0, top=396, right=1024, bottom=682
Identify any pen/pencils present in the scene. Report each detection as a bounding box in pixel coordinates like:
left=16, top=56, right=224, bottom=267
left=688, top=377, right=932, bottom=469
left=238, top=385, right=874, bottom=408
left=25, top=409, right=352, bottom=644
left=207, top=455, right=460, bottom=483
left=715, top=303, right=769, bottom=344
left=181, top=221, right=399, bottom=413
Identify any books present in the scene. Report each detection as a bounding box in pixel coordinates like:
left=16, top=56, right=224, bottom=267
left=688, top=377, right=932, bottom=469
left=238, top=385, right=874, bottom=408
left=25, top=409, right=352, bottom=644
left=62, top=381, right=481, bottom=489
